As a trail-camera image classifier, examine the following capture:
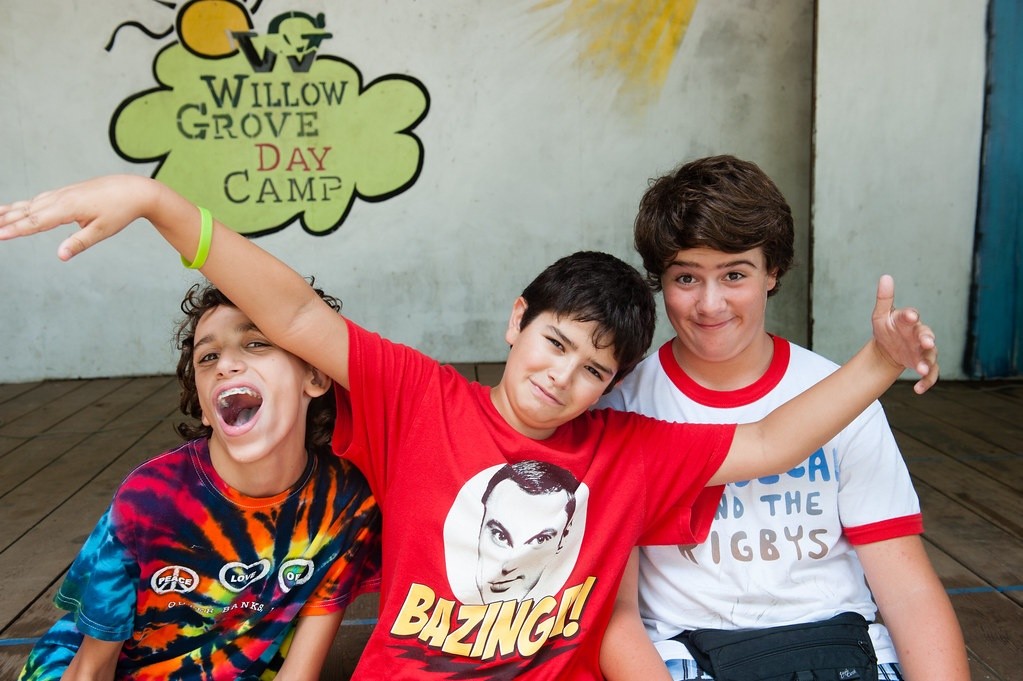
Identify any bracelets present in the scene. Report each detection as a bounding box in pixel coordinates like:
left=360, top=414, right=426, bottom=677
left=181, top=206, right=212, bottom=270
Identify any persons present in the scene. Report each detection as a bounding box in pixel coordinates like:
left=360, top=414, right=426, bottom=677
left=591, top=154, right=972, bottom=681
left=19, top=277, right=381, bottom=681
left=0, top=174, right=938, bottom=681
